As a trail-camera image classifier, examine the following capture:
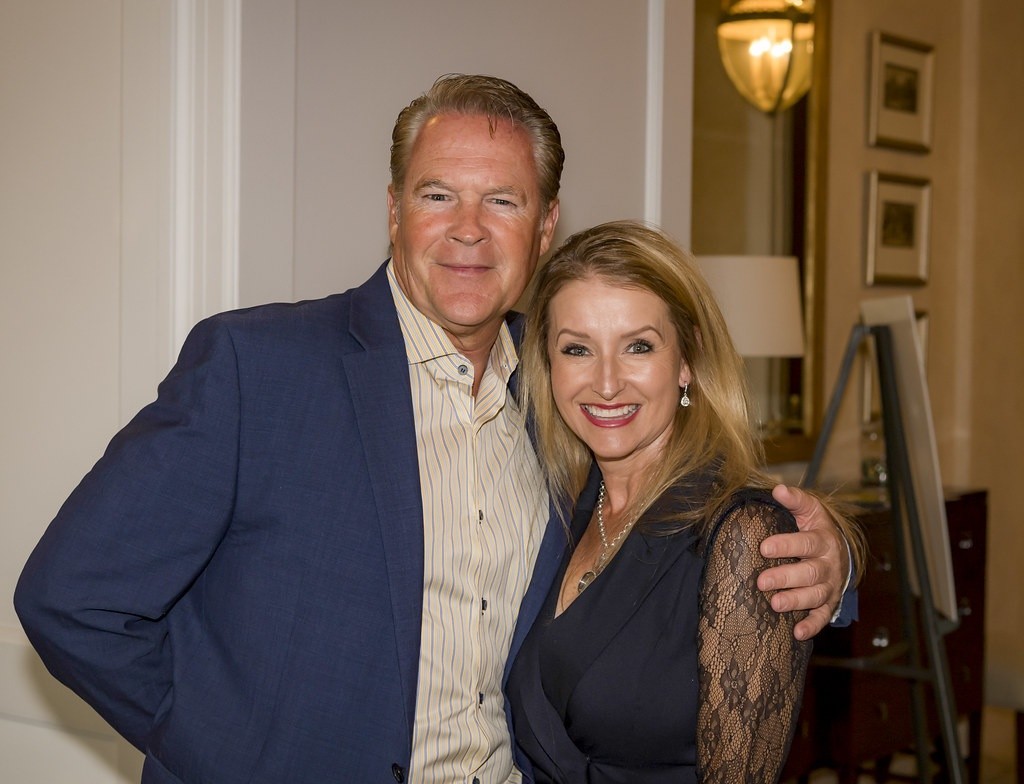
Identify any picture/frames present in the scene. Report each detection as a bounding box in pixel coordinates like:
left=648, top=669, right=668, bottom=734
left=863, top=168, right=935, bottom=287
left=867, top=26, right=938, bottom=156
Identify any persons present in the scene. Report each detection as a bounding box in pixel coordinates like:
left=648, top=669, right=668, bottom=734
left=502, top=221, right=813, bottom=784
left=14, top=75, right=863, bottom=784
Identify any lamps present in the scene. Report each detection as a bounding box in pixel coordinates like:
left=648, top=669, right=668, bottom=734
left=715, top=0, right=819, bottom=116
left=687, top=255, right=806, bottom=362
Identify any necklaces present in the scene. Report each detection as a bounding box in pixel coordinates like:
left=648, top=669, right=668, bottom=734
left=578, top=480, right=651, bottom=593
